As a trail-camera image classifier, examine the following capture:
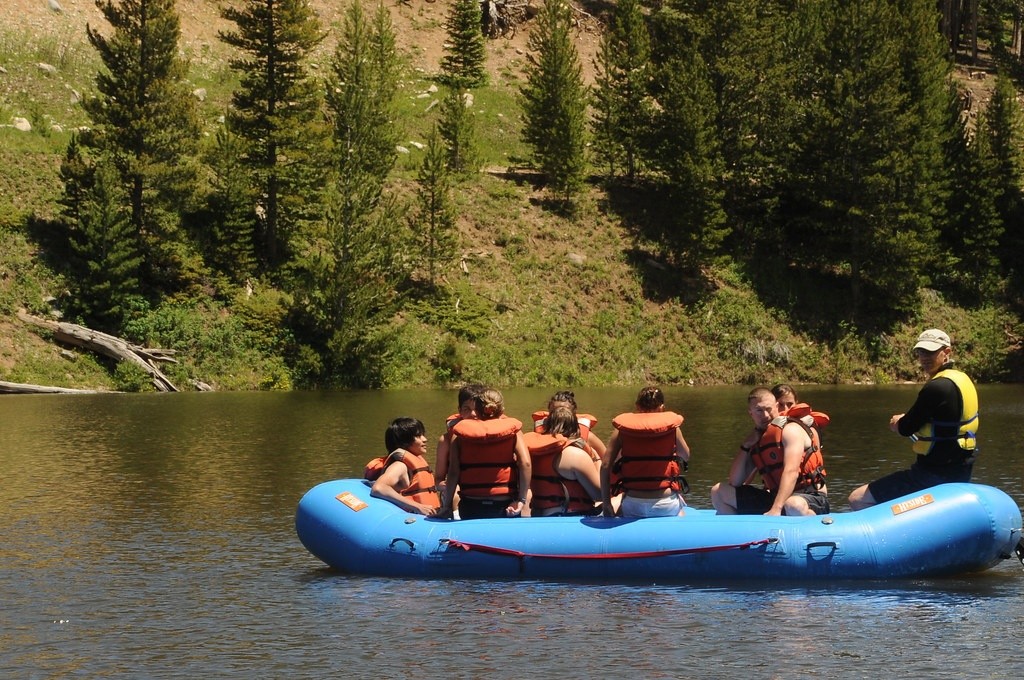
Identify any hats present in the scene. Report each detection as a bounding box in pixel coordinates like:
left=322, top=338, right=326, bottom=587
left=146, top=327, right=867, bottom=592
left=913, top=329, right=950, bottom=352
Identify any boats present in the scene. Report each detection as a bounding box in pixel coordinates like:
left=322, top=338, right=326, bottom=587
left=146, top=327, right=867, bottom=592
left=294, top=477, right=1024, bottom=589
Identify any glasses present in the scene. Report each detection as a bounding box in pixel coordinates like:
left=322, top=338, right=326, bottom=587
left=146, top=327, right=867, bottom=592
left=917, top=346, right=944, bottom=358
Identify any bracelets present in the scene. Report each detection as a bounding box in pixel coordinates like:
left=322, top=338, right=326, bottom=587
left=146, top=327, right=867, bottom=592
left=740, top=445, right=750, bottom=452
left=519, top=497, right=526, bottom=505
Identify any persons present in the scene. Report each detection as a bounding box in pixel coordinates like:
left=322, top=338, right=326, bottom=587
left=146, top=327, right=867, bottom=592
left=364, top=418, right=460, bottom=516
left=434, top=385, right=507, bottom=490
left=711, top=387, right=830, bottom=516
left=532, top=390, right=607, bottom=461
left=524, top=406, right=623, bottom=517
left=600, top=386, right=690, bottom=518
left=848, top=328, right=979, bottom=511
left=428, top=389, right=531, bottom=520
left=771, top=383, right=830, bottom=449
